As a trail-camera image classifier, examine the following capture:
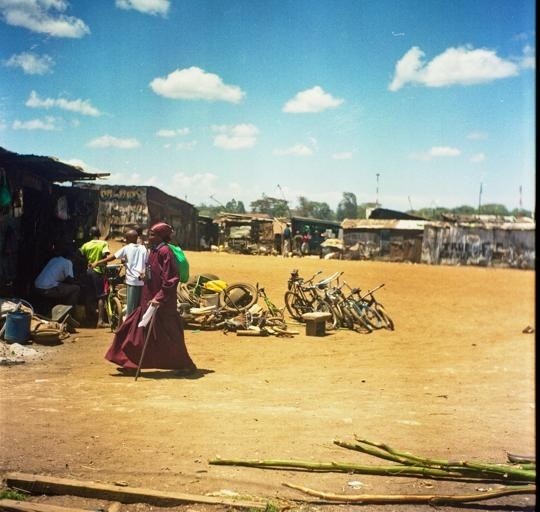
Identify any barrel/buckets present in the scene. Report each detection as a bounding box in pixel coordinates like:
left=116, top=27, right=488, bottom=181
left=4, top=311, right=32, bottom=345
left=199, top=292, right=220, bottom=307
left=118, top=288, right=127, bottom=304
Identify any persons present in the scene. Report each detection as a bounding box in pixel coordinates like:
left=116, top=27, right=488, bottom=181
left=88, top=228, right=149, bottom=319
left=33, top=248, right=82, bottom=309
left=300, top=231, right=312, bottom=254
left=282, top=222, right=292, bottom=259
left=293, top=229, right=304, bottom=256
left=200, top=234, right=209, bottom=249
left=78, top=224, right=112, bottom=329
left=318, top=231, right=330, bottom=261
left=103, top=221, right=198, bottom=378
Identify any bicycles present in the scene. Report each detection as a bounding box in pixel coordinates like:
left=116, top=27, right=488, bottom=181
left=254, top=267, right=394, bottom=337
left=97, top=258, right=127, bottom=334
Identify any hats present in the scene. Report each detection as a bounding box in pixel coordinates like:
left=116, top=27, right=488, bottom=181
left=151, top=222, right=174, bottom=240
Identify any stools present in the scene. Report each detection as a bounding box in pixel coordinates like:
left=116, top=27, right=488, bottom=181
left=301, top=310, right=332, bottom=338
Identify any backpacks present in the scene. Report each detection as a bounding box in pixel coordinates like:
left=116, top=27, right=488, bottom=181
left=168, top=242, right=190, bottom=285
